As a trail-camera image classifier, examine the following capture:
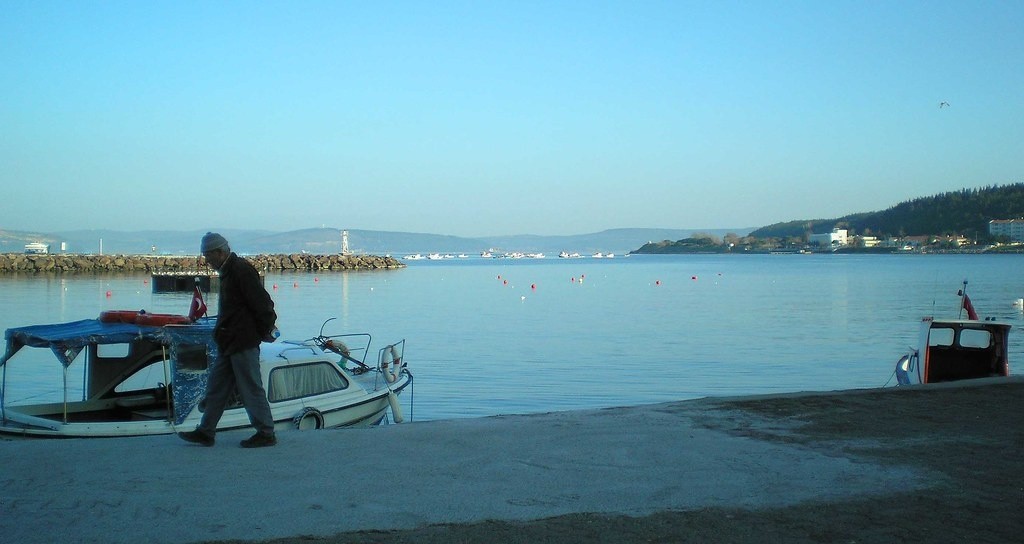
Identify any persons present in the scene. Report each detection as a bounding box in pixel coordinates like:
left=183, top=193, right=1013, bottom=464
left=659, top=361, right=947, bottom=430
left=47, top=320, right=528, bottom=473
left=176, top=231, right=278, bottom=448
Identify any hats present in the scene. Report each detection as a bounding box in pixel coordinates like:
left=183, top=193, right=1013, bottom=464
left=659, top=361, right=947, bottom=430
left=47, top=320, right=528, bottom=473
left=198, top=229, right=228, bottom=252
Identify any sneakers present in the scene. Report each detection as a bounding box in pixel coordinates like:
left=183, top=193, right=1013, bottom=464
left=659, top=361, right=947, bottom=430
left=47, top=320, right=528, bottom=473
left=177, top=430, right=215, bottom=447
left=241, top=432, right=277, bottom=447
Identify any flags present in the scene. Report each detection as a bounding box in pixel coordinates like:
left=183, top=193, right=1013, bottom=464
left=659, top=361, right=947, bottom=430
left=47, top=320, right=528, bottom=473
left=186, top=285, right=207, bottom=322
left=962, top=294, right=979, bottom=321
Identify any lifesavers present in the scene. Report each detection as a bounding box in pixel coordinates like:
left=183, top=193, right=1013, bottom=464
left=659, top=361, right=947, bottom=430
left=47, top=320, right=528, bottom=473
left=293, top=406, right=325, bottom=430
left=318, top=339, right=349, bottom=368
left=381, top=345, right=400, bottom=383
left=99, top=310, right=191, bottom=325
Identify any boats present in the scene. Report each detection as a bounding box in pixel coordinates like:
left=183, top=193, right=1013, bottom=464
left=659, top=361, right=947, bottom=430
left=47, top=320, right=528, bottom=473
left=0, top=272, right=416, bottom=437
left=895, top=269, right=1014, bottom=386
